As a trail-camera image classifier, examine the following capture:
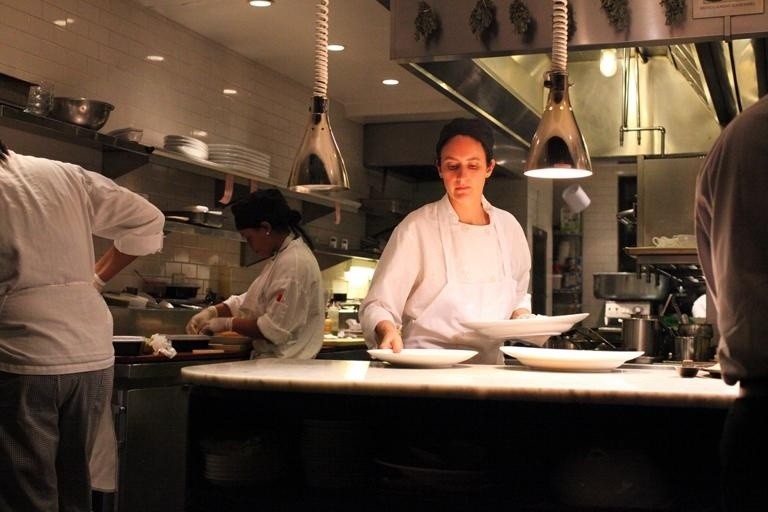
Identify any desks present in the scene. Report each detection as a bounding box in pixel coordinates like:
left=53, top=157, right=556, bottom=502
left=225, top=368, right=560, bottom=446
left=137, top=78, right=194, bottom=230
left=175, top=350, right=743, bottom=511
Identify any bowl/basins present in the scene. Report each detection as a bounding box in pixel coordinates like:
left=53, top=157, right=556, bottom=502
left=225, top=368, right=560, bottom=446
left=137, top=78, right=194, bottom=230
left=48, top=97, right=116, bottom=131
left=674, top=337, right=710, bottom=361
left=143, top=283, right=169, bottom=297
left=113, top=334, right=209, bottom=355
left=678, top=323, right=714, bottom=338
left=166, top=285, right=201, bottom=299
left=591, top=272, right=671, bottom=302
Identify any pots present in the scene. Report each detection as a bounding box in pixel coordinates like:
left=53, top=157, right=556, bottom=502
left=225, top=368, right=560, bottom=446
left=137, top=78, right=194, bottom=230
left=620, top=318, right=663, bottom=358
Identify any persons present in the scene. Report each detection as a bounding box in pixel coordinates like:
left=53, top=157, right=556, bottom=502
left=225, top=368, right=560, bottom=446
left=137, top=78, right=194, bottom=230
left=185, top=189, right=326, bottom=360
left=358, top=119, right=550, bottom=368
left=0, top=136, right=166, bottom=512
left=693, top=92, right=768, bottom=512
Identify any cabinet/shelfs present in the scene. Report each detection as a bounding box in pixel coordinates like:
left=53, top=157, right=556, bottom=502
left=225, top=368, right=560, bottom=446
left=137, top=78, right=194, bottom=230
left=551, top=228, right=585, bottom=304
left=0, top=100, right=360, bottom=268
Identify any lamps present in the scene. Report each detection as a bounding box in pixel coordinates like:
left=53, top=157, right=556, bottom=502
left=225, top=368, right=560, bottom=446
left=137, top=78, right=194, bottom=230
left=284, top=1, right=352, bottom=192
left=521, top=2, right=595, bottom=177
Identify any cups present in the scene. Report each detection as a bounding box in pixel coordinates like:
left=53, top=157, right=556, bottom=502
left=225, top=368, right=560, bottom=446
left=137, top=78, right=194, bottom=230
left=28, top=79, right=54, bottom=116
left=651, top=236, right=677, bottom=247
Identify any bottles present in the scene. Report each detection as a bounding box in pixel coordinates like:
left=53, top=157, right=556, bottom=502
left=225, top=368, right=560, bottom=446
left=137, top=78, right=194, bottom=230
left=567, top=257, right=576, bottom=271
left=327, top=301, right=339, bottom=331
left=554, top=258, right=561, bottom=274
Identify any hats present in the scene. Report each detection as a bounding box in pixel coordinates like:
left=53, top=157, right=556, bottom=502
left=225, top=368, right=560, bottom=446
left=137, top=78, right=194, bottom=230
left=231, top=188, right=301, bottom=230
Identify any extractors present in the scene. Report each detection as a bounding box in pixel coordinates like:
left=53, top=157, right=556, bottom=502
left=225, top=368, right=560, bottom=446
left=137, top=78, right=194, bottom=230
left=374, top=1, right=767, bottom=168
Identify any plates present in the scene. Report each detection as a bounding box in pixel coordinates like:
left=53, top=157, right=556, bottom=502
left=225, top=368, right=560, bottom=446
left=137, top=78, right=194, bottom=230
left=365, top=348, right=478, bottom=369
left=164, top=135, right=272, bottom=178
left=180, top=204, right=224, bottom=215
left=499, top=345, right=645, bottom=372
left=458, top=312, right=590, bottom=340
left=106, top=128, right=143, bottom=142
